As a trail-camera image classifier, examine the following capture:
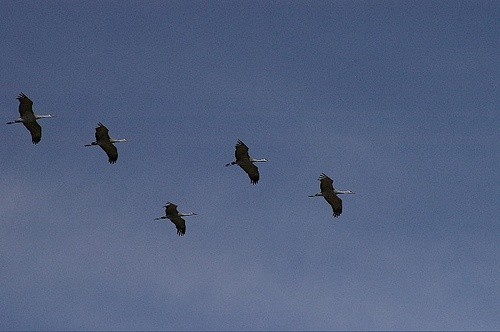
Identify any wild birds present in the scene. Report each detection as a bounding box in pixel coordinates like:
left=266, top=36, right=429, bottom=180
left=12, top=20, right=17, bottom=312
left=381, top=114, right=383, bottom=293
left=6, top=92, right=54, bottom=145
left=225, top=139, right=269, bottom=186
left=308, top=172, right=355, bottom=218
left=153, top=201, right=198, bottom=237
left=84, top=121, right=128, bottom=164
